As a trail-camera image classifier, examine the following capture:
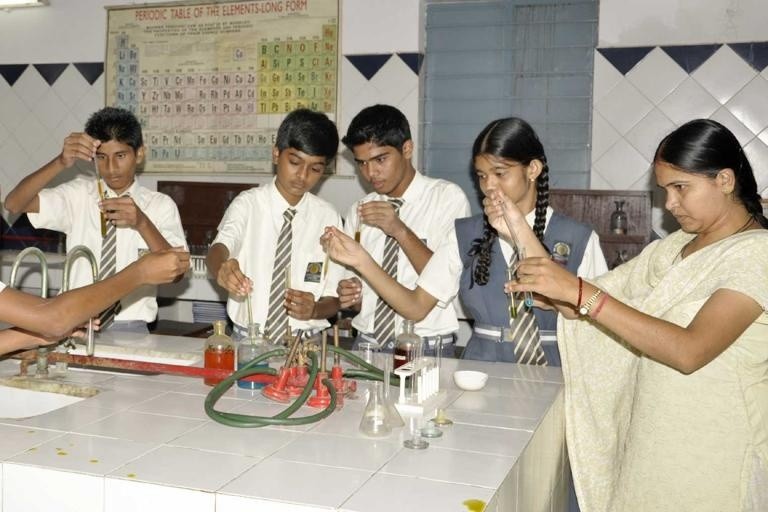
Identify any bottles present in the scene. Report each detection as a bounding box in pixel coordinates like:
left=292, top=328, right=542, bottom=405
left=201, top=317, right=236, bottom=389
left=284, top=263, right=294, bottom=315
left=353, top=199, right=365, bottom=243
left=234, top=320, right=271, bottom=390
left=504, top=247, right=534, bottom=321
left=86, top=316, right=95, bottom=357
left=359, top=318, right=445, bottom=439
left=98, top=208, right=108, bottom=241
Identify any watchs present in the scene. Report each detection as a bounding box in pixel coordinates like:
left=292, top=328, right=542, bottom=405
left=579, top=287, right=603, bottom=316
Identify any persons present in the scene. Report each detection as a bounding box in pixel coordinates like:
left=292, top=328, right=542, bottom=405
left=4, top=105, right=190, bottom=334
left=320, top=117, right=610, bottom=368
left=201, top=106, right=344, bottom=349
left=481, top=119, right=768, bottom=512
left=0, top=245, right=192, bottom=359
left=335, top=104, right=472, bottom=359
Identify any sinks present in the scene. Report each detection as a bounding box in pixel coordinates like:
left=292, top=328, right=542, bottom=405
left=52, top=345, right=201, bottom=375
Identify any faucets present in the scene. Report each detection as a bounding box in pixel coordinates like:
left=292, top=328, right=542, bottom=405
left=61, top=244, right=98, bottom=293
left=10, top=247, right=48, bottom=300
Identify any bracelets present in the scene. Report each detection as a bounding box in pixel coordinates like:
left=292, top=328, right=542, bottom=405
left=575, top=275, right=583, bottom=312
left=589, top=292, right=608, bottom=322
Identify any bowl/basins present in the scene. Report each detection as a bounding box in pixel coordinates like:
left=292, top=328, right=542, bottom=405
left=451, top=369, right=489, bottom=391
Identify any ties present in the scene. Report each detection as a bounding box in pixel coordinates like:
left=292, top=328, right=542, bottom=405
left=97, top=189, right=117, bottom=328
left=264, top=206, right=296, bottom=343
left=504, top=239, right=550, bottom=367
left=370, top=197, right=403, bottom=350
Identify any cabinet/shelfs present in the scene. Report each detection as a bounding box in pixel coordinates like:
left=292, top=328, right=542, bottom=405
left=549, top=187, right=654, bottom=270
left=158, top=182, right=258, bottom=256
